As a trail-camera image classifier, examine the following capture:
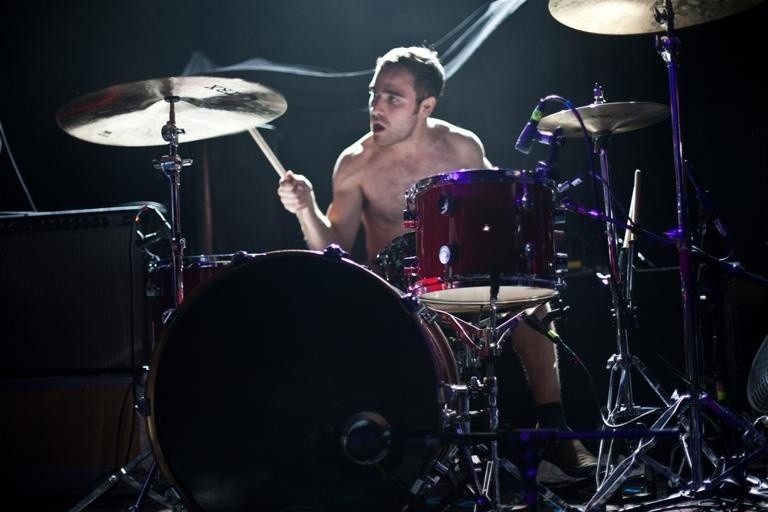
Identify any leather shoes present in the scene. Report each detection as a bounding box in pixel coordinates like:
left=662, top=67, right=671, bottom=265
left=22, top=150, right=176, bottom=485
left=533, top=423, right=598, bottom=474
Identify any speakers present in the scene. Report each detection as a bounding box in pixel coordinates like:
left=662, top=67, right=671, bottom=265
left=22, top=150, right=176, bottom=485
left=1, top=202, right=167, bottom=380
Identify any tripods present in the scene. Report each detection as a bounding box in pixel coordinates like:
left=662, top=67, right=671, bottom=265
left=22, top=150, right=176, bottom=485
left=472, top=349, right=574, bottom=511
left=582, top=26, right=768, bottom=512
left=71, top=236, right=173, bottom=512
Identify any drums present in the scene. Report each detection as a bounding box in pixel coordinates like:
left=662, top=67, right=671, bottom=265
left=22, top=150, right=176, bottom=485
left=407, top=166, right=561, bottom=308
left=142, top=248, right=462, bottom=512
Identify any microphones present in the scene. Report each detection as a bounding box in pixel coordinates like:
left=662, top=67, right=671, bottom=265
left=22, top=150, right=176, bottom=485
left=515, top=101, right=551, bottom=155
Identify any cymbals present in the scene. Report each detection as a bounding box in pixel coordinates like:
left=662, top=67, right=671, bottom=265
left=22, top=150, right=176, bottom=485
left=56, top=77, right=287, bottom=147
left=538, top=102, right=671, bottom=139
left=548, top=0, right=764, bottom=34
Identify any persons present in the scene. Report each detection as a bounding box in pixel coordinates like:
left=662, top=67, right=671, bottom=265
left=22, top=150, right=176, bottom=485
left=274, top=45, right=600, bottom=481
